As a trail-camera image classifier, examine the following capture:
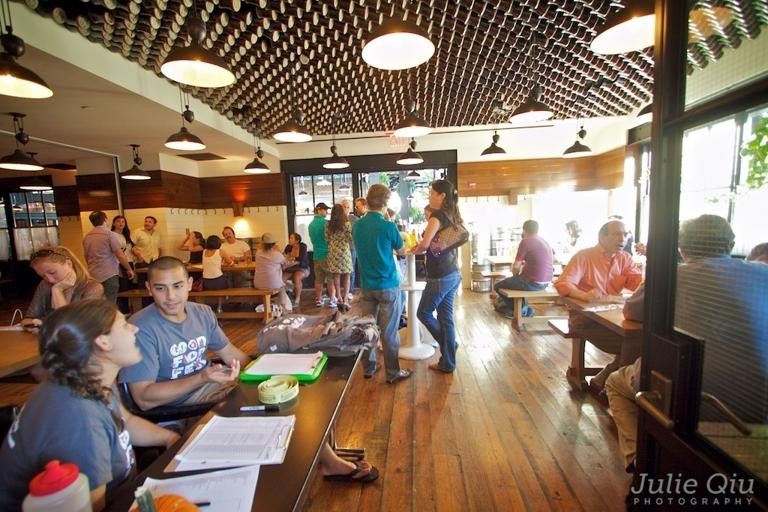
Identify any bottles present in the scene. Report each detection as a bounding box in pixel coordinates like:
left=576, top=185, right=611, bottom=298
left=19, top=460, right=93, bottom=510
left=408, top=229, right=417, bottom=247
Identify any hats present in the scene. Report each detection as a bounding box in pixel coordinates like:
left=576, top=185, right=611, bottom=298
left=260, top=232, right=280, bottom=244
left=316, top=202, right=331, bottom=209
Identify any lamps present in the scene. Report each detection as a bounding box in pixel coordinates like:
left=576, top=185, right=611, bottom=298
left=562, top=119, right=592, bottom=155
left=589, top=2, right=657, bottom=55
left=0, top=0, right=54, bottom=100
left=1, top=112, right=53, bottom=192
left=362, top=13, right=436, bottom=72
left=392, top=99, right=433, bottom=179
left=243, top=118, right=271, bottom=174
left=162, top=83, right=206, bottom=151
left=231, top=202, right=245, bottom=217
left=160, top=0, right=238, bottom=89
left=479, top=131, right=506, bottom=156
left=269, top=105, right=314, bottom=143
left=298, top=176, right=308, bottom=195
left=119, top=143, right=151, bottom=181
left=338, top=174, right=351, bottom=190
left=322, top=141, right=350, bottom=169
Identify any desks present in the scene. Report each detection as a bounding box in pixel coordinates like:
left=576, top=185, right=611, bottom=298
left=0, top=330, right=43, bottom=385
left=95, top=299, right=380, bottom=511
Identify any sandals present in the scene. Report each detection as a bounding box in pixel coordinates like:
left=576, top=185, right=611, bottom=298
left=322, top=460, right=380, bottom=484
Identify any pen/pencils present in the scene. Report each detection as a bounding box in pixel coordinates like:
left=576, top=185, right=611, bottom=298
left=195, top=502, right=211, bottom=506
left=240, top=405, right=280, bottom=412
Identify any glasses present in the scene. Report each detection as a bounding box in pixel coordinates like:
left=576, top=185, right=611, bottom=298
left=30, top=248, right=65, bottom=261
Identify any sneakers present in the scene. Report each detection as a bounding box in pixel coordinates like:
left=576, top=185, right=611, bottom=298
left=429, top=362, right=454, bottom=373
left=292, top=302, right=300, bottom=308
left=385, top=367, right=414, bottom=384
left=590, top=379, right=610, bottom=407
left=522, top=303, right=535, bottom=317
left=313, top=293, right=354, bottom=313
left=363, top=361, right=382, bottom=379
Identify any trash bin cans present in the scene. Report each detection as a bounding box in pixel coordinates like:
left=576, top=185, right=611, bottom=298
left=471, top=263, right=491, bottom=292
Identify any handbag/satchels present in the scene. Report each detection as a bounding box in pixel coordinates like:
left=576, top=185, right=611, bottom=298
left=429, top=209, right=470, bottom=258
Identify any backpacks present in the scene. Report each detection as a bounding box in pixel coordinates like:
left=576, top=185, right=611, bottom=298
left=255, top=313, right=381, bottom=357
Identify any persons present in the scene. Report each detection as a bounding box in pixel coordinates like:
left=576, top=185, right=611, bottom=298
left=608, top=215, right=633, bottom=256
left=554, top=221, right=647, bottom=401
left=495, top=220, right=554, bottom=318
left=0, top=179, right=462, bottom=512
left=556, top=221, right=584, bottom=275
left=605, top=215, right=768, bottom=477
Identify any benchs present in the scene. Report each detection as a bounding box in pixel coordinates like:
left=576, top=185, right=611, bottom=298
left=114, top=259, right=292, bottom=327
left=481, top=254, right=644, bottom=432
